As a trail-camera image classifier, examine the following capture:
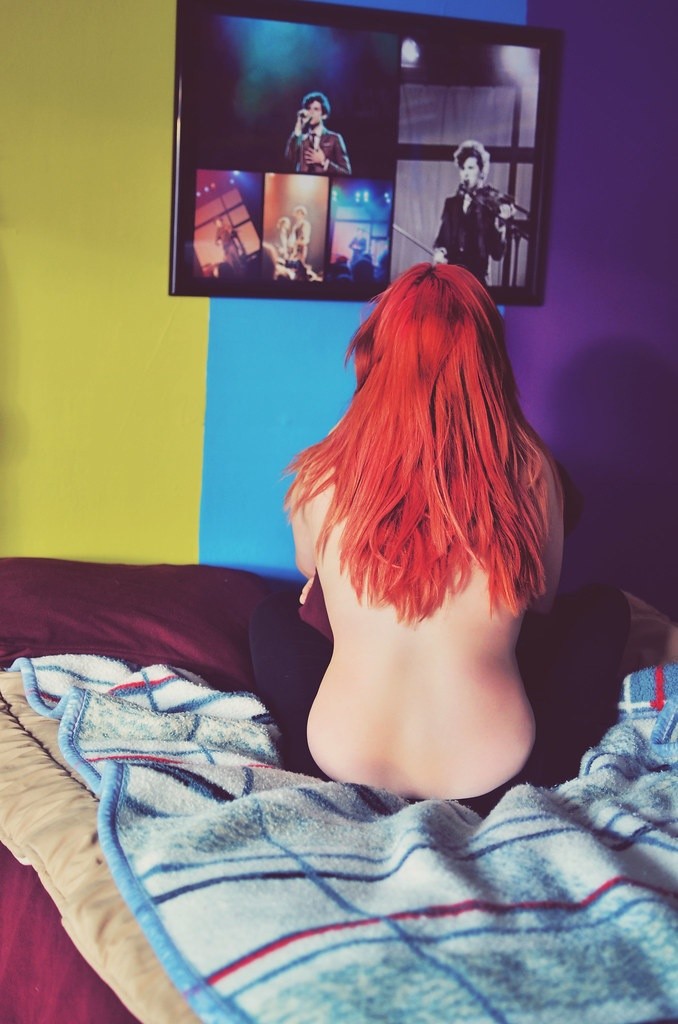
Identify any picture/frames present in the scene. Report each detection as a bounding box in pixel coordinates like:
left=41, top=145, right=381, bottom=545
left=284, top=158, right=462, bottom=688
left=170, top=4, right=561, bottom=309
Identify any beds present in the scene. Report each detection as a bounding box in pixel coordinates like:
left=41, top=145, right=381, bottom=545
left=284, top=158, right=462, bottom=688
left=0, top=557, right=676, bottom=1024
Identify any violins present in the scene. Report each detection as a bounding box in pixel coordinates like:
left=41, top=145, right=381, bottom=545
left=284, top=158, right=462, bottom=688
left=475, top=185, right=529, bottom=217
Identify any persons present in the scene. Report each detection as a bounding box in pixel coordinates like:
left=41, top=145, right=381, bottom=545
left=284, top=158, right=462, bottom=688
left=432, top=140, right=516, bottom=284
left=247, top=261, right=632, bottom=820
left=210, top=207, right=388, bottom=284
left=283, top=91, right=352, bottom=178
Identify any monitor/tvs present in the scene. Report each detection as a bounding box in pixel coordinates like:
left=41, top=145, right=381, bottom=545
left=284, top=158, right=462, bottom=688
left=168, top=0, right=558, bottom=309
